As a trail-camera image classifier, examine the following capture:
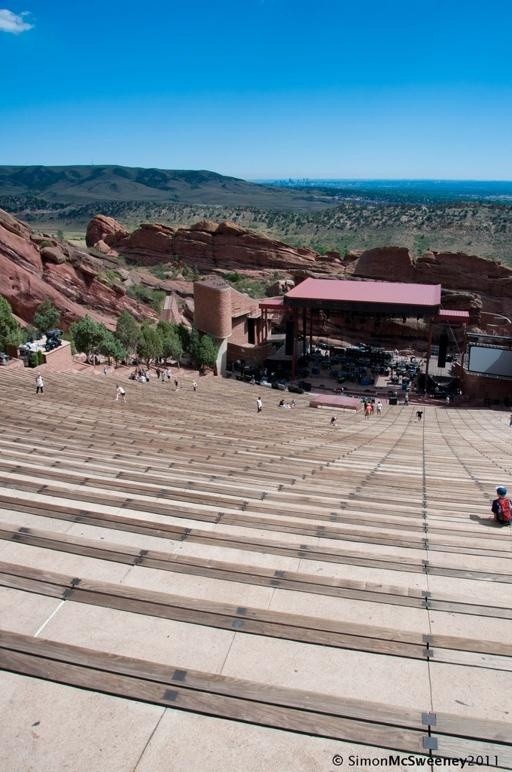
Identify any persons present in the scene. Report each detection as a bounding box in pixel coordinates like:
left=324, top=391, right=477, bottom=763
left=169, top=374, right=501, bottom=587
left=291, top=399, right=296, bottom=409
left=128, top=366, right=198, bottom=391
left=416, top=409, right=423, bottom=422
left=279, top=399, right=285, bottom=407
left=35, top=371, right=45, bottom=397
left=256, top=396, right=263, bottom=414
left=103, top=365, right=107, bottom=376
left=403, top=392, right=409, bottom=406
left=330, top=416, right=336, bottom=426
left=490, top=485, right=511, bottom=526
left=364, top=400, right=382, bottom=422
left=114, top=384, right=127, bottom=401
left=286, top=402, right=291, bottom=408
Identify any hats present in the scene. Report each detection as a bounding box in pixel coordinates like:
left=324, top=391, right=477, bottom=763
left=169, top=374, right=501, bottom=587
left=495, top=486, right=507, bottom=496
